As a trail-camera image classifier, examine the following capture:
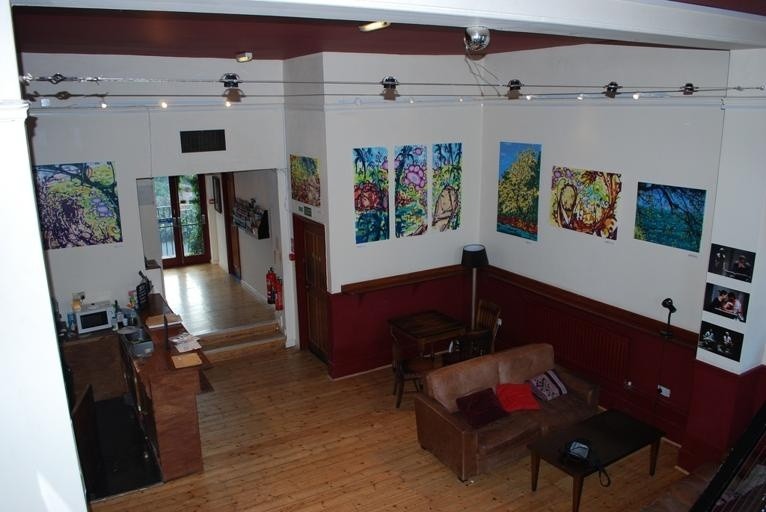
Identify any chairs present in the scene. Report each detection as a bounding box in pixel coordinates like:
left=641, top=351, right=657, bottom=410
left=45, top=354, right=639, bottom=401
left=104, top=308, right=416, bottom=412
left=385, top=308, right=501, bottom=407
left=71, top=383, right=99, bottom=468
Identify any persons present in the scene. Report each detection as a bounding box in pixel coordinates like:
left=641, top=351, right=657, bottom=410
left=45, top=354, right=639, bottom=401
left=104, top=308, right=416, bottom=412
left=710, top=289, right=734, bottom=312
left=701, top=327, right=716, bottom=346
left=713, top=247, right=726, bottom=273
left=730, top=253, right=753, bottom=283
left=726, top=292, right=742, bottom=315
left=720, top=331, right=734, bottom=352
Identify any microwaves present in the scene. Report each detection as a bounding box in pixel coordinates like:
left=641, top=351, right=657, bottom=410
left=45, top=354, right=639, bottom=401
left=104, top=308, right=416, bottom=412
left=74, top=299, right=113, bottom=335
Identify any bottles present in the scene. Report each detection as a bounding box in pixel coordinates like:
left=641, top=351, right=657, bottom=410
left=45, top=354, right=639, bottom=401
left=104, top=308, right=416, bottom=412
left=111, top=317, right=118, bottom=331
left=68, top=312, right=77, bottom=331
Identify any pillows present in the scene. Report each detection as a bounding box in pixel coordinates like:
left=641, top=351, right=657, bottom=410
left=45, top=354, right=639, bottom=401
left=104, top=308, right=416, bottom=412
left=455, top=368, right=570, bottom=429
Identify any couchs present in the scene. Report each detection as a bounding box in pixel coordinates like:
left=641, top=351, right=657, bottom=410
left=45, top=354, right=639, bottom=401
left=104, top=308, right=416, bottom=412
left=415, top=341, right=595, bottom=483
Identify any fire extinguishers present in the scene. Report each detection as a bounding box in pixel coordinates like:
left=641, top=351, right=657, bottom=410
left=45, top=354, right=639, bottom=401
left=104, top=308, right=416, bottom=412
left=266, top=267, right=283, bottom=310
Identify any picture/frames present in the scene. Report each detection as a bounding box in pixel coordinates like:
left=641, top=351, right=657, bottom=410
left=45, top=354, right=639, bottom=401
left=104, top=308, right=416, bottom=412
left=212, top=176, right=221, bottom=213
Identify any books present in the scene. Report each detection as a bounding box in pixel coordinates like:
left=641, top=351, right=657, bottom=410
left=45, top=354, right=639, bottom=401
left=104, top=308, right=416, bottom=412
left=170, top=353, right=203, bottom=369
left=174, top=341, right=202, bottom=352
left=146, top=314, right=164, bottom=328
left=168, top=332, right=200, bottom=343
left=164, top=313, right=183, bottom=325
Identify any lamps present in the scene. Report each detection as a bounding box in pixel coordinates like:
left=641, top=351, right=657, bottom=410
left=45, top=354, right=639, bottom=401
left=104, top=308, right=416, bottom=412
left=236, top=50, right=253, bottom=63
left=604, top=81, right=624, bottom=97
left=221, top=72, right=245, bottom=103
left=505, top=79, right=523, bottom=100
left=660, top=297, right=677, bottom=336
left=461, top=244, right=489, bottom=331
left=356, top=21, right=390, bottom=32
left=684, top=82, right=694, bottom=95
left=379, top=76, right=400, bottom=100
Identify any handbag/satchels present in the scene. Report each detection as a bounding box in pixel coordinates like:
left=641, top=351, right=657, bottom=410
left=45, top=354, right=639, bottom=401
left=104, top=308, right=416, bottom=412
left=566, top=439, right=593, bottom=463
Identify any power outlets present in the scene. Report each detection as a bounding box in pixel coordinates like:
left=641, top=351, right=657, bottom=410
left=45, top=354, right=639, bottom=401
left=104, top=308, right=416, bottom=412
left=657, top=383, right=671, bottom=399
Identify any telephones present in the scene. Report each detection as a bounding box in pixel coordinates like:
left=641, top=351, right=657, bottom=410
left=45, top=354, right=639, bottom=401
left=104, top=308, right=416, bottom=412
left=565, top=437, right=593, bottom=464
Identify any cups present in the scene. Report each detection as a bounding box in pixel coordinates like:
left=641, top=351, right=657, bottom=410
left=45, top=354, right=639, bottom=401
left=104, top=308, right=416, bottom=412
left=123, top=318, right=129, bottom=327
